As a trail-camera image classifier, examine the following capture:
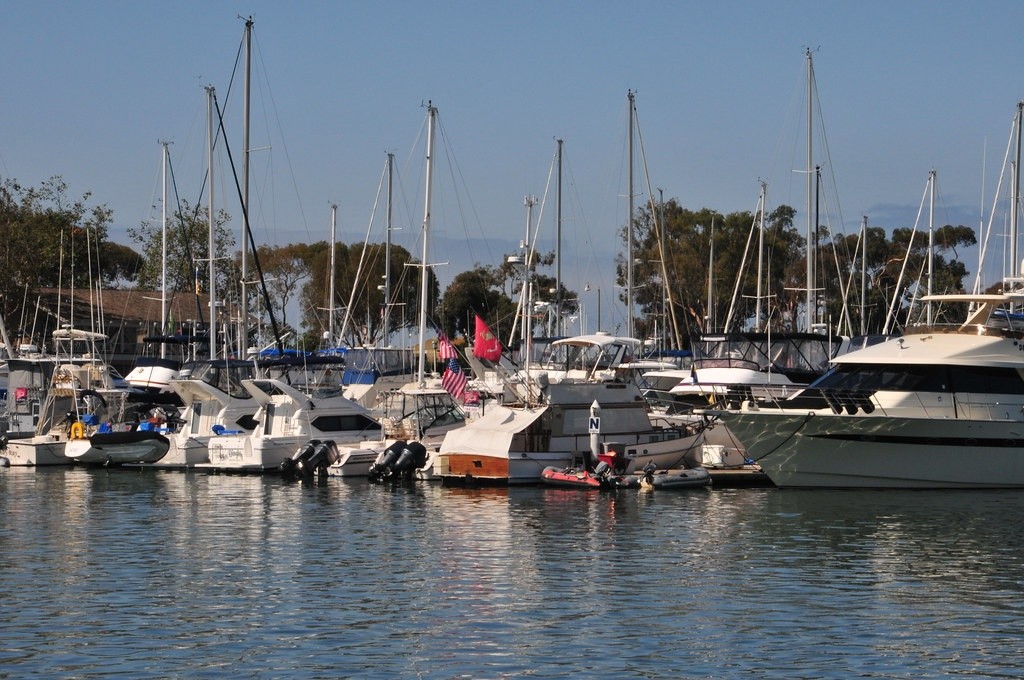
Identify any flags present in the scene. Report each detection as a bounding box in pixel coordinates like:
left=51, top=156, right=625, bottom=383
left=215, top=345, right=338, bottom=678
left=441, top=357, right=468, bottom=401
left=472, top=314, right=504, bottom=364
left=436, top=329, right=459, bottom=360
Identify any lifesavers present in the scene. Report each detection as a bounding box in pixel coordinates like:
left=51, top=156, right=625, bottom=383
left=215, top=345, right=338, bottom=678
left=70, top=422, right=83, bottom=438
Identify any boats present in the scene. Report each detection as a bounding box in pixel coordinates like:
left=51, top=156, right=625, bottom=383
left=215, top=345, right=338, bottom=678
left=0, top=9, right=1024, bottom=492
